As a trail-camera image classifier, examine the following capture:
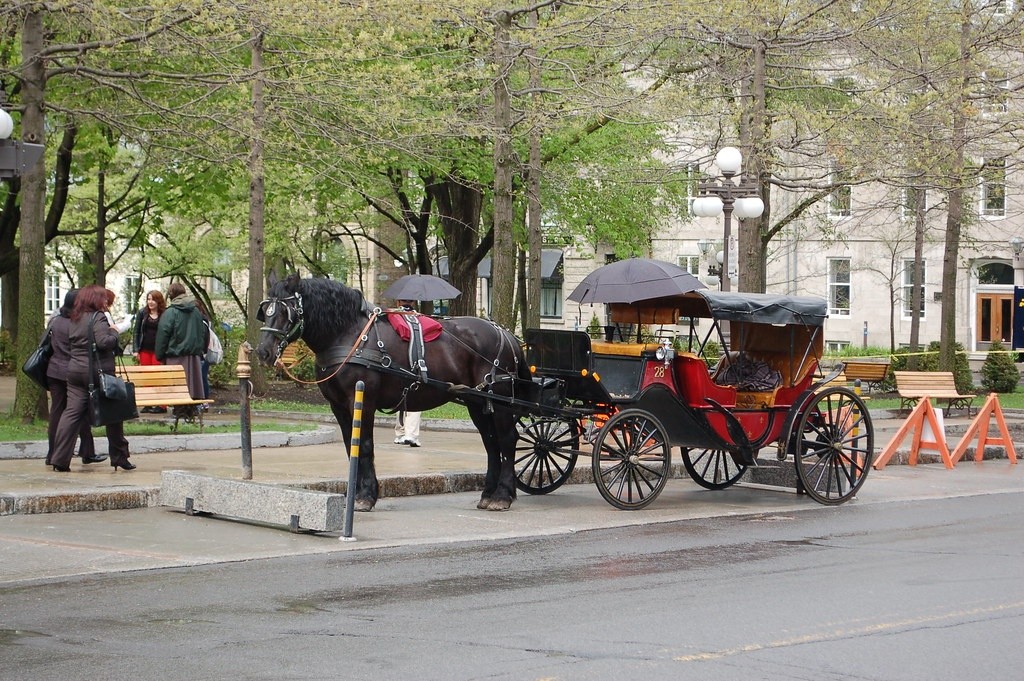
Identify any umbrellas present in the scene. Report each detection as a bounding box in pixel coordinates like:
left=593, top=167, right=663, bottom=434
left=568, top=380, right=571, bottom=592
left=564, top=257, right=709, bottom=306
left=384, top=274, right=462, bottom=301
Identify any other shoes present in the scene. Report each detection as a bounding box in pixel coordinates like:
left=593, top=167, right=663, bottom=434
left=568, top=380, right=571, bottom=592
left=394, top=436, right=405, bottom=444
left=404, top=437, right=421, bottom=447
left=140, top=406, right=152, bottom=413
left=148, top=406, right=167, bottom=413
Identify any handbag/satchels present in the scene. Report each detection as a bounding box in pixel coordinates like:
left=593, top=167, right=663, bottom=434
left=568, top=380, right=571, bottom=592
left=89, top=382, right=139, bottom=426
left=22, top=316, right=62, bottom=390
left=98, top=373, right=128, bottom=400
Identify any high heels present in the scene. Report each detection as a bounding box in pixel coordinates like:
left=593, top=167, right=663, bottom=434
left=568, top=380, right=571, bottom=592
left=52, top=464, right=71, bottom=472
left=114, top=460, right=136, bottom=471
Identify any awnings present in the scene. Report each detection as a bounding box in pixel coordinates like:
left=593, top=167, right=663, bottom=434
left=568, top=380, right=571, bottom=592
left=432, top=251, right=562, bottom=282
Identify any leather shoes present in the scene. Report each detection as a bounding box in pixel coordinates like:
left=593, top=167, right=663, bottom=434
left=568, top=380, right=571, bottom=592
left=45, top=459, right=52, bottom=465
left=82, top=455, right=107, bottom=464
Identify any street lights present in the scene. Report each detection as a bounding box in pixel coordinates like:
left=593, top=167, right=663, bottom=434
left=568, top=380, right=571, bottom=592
left=692, top=145, right=765, bottom=357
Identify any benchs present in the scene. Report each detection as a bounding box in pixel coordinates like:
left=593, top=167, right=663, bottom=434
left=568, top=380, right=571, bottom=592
left=842, top=361, right=891, bottom=396
left=893, top=370, right=978, bottom=421
left=47, top=365, right=216, bottom=436
left=673, top=349, right=737, bottom=409
left=811, top=371, right=871, bottom=410
left=711, top=350, right=819, bottom=408
left=524, top=328, right=594, bottom=402
left=586, top=337, right=675, bottom=398
left=279, top=342, right=315, bottom=380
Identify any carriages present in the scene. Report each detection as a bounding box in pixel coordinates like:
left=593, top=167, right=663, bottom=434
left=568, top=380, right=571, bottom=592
left=257, top=254, right=878, bottom=512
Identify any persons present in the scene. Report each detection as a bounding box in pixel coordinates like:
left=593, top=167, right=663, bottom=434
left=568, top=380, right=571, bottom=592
left=45, top=284, right=136, bottom=472
left=132, top=290, right=167, bottom=413
left=394, top=300, right=421, bottom=447
left=155, top=283, right=206, bottom=417
left=190, top=300, right=210, bottom=412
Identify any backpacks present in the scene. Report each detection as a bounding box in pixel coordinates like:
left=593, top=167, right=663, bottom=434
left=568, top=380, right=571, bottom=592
left=203, top=320, right=224, bottom=364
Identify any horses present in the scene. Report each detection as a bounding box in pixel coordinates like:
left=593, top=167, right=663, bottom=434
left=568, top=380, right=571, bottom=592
left=256, top=269, right=534, bottom=513
